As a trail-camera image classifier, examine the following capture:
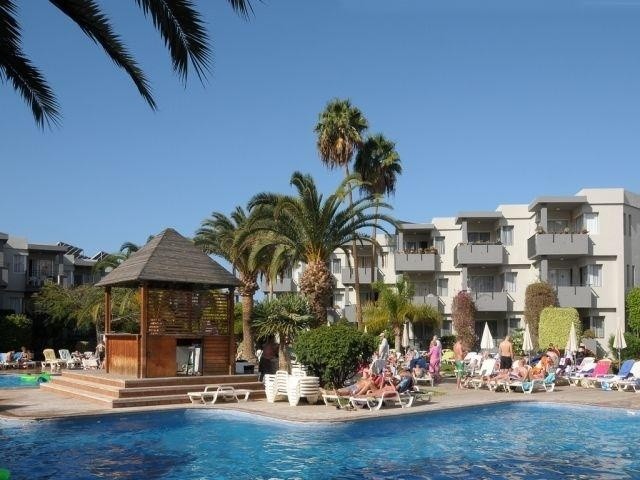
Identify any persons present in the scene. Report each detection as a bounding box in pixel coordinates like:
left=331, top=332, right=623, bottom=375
left=15, top=346, right=29, bottom=368
left=316, top=331, right=573, bottom=399
left=74, top=349, right=85, bottom=365
left=203, top=319, right=219, bottom=337
left=95, top=339, right=105, bottom=368
left=26, top=350, right=32, bottom=362
left=257, top=334, right=280, bottom=383
left=149, top=312, right=166, bottom=334
left=5, top=350, right=16, bottom=370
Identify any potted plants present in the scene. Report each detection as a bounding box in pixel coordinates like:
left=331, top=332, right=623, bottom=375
left=403, top=247, right=438, bottom=254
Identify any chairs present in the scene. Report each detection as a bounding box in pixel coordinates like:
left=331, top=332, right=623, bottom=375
left=263, top=367, right=320, bottom=406
left=187, top=384, right=252, bottom=405
left=458, top=351, right=557, bottom=395
left=0, top=346, right=99, bottom=374
left=559, top=356, right=639, bottom=392
left=321, top=347, right=435, bottom=410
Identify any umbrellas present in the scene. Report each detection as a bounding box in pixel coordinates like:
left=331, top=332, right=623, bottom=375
left=564, top=321, right=584, bottom=367
left=521, top=323, right=535, bottom=362
left=400, top=321, right=410, bottom=356
left=479, top=321, right=496, bottom=355
left=612, top=317, right=629, bottom=372
